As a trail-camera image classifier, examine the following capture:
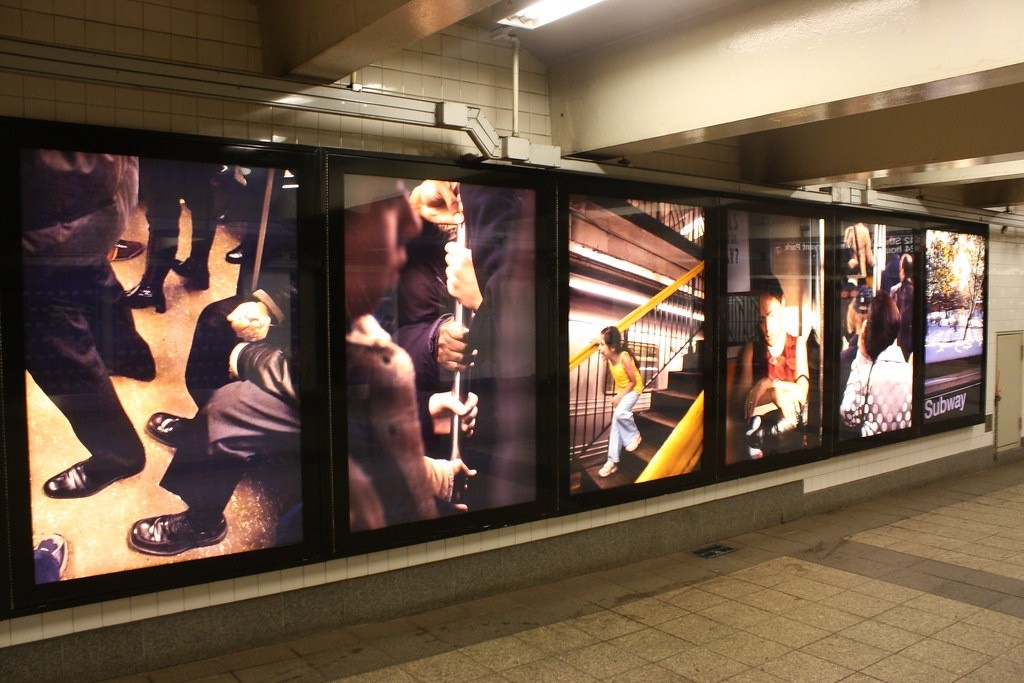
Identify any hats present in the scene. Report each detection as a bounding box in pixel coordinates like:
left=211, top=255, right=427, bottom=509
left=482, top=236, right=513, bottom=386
left=854, top=285, right=872, bottom=313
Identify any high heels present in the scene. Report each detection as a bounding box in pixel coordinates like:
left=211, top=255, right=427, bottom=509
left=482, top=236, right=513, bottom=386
left=125, top=281, right=167, bottom=313
left=172, top=257, right=210, bottom=290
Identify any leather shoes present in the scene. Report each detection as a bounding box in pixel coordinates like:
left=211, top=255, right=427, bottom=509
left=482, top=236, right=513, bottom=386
left=226, top=244, right=243, bottom=263
left=43, top=459, right=145, bottom=498
left=146, top=411, right=192, bottom=447
left=130, top=514, right=228, bottom=555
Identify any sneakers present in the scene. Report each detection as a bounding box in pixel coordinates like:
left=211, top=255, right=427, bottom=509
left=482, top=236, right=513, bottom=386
left=106, top=239, right=143, bottom=261
left=625, top=431, right=642, bottom=452
left=34, top=532, right=68, bottom=578
left=598, top=461, right=618, bottom=478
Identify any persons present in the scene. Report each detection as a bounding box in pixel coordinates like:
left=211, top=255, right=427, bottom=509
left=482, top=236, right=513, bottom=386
left=840, top=223, right=913, bottom=437
left=729, top=273, right=810, bottom=462
left=343, top=173, right=537, bottom=531
left=599, top=326, right=643, bottom=477
left=19, top=148, right=300, bottom=584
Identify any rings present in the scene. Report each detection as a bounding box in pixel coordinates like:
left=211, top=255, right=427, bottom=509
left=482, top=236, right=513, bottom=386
left=750, top=402, right=753, bottom=404
